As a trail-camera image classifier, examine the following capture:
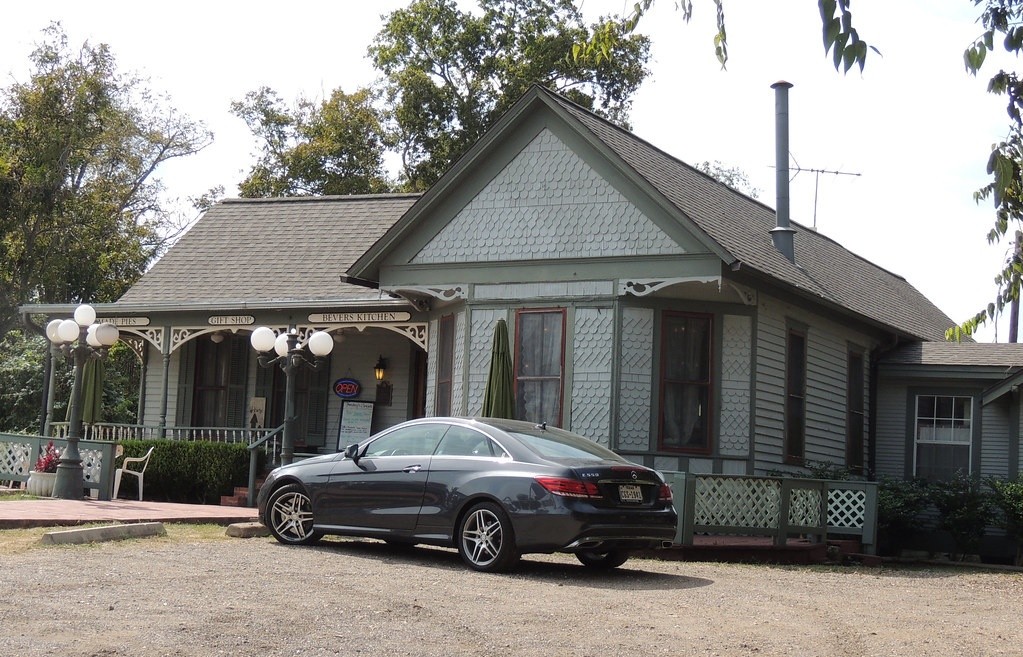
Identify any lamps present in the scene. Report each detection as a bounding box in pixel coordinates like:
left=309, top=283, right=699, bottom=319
left=373, top=354, right=387, bottom=381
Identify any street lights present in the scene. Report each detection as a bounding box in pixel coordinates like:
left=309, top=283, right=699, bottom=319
left=46, top=302, right=119, bottom=500
left=252, top=326, right=334, bottom=466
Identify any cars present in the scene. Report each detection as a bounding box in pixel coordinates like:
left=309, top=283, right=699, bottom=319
left=255, top=416, right=678, bottom=573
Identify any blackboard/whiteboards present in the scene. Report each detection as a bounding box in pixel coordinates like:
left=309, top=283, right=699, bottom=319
left=336, top=398, right=376, bottom=452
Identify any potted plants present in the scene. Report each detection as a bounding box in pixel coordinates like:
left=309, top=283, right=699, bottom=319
left=27, top=440, right=61, bottom=497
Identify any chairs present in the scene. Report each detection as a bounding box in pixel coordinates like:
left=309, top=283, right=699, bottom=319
left=113, top=447, right=154, bottom=501
